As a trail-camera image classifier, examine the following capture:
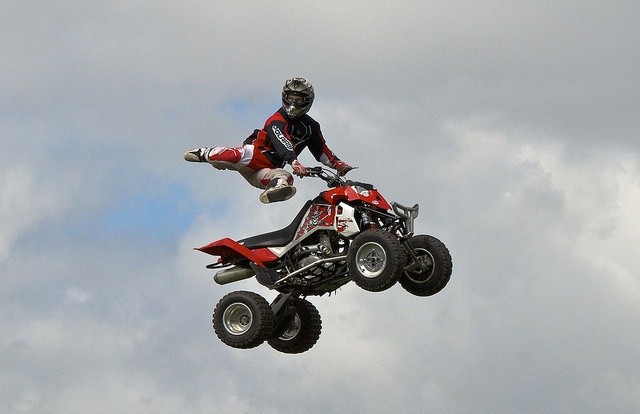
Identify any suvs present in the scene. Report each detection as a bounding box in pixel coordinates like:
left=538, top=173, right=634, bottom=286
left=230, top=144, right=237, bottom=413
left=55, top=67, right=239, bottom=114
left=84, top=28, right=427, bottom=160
left=193, top=165, right=453, bottom=354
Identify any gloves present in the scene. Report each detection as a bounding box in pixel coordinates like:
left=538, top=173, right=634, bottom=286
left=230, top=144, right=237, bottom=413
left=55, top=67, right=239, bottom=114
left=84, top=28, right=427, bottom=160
left=292, top=160, right=307, bottom=179
left=336, top=162, right=352, bottom=176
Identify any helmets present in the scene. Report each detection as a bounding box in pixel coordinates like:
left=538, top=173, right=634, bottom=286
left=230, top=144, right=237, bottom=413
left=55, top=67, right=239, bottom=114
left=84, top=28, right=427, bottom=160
left=282, top=78, right=314, bottom=118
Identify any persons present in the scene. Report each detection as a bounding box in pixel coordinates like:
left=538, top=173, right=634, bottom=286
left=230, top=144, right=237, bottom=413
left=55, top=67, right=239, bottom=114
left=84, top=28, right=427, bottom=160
left=184, top=77, right=352, bottom=204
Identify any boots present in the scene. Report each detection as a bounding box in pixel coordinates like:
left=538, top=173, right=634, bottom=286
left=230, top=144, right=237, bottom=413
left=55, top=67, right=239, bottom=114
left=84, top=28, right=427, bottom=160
left=260, top=178, right=296, bottom=204
left=184, top=147, right=214, bottom=162
